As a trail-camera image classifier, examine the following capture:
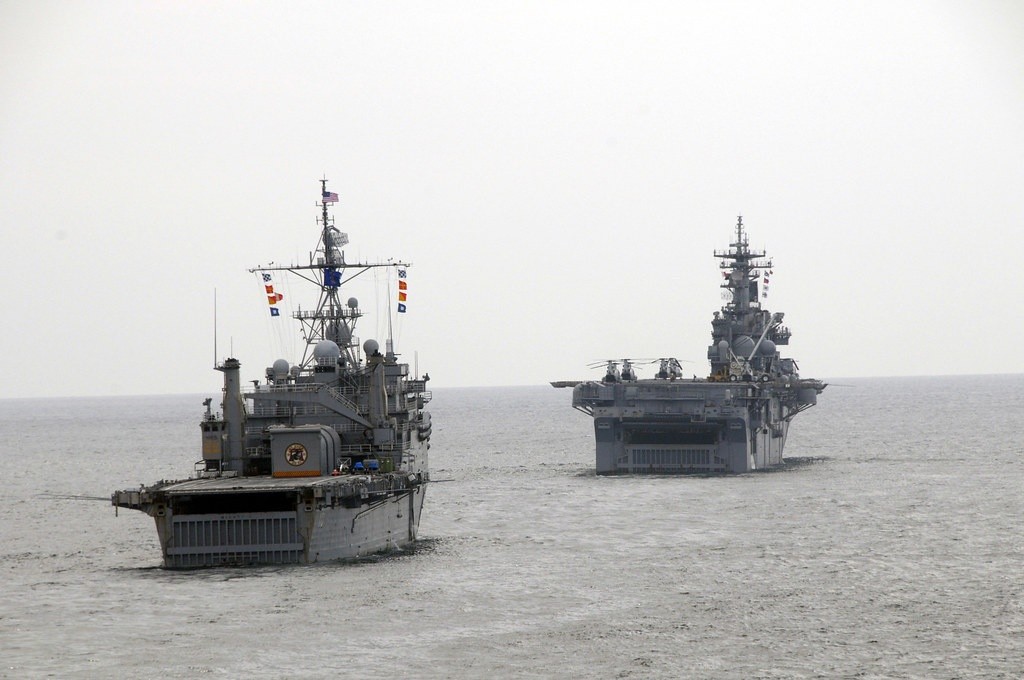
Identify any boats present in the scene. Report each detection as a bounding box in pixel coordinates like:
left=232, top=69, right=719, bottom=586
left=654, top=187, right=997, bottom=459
left=572, top=216, right=831, bottom=474
left=113, top=173, right=433, bottom=567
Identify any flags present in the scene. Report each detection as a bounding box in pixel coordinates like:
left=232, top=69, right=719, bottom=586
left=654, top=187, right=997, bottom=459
left=762, top=270, right=773, bottom=298
left=323, top=192, right=339, bottom=202
left=398, top=270, right=407, bottom=312
left=262, top=273, right=283, bottom=316
